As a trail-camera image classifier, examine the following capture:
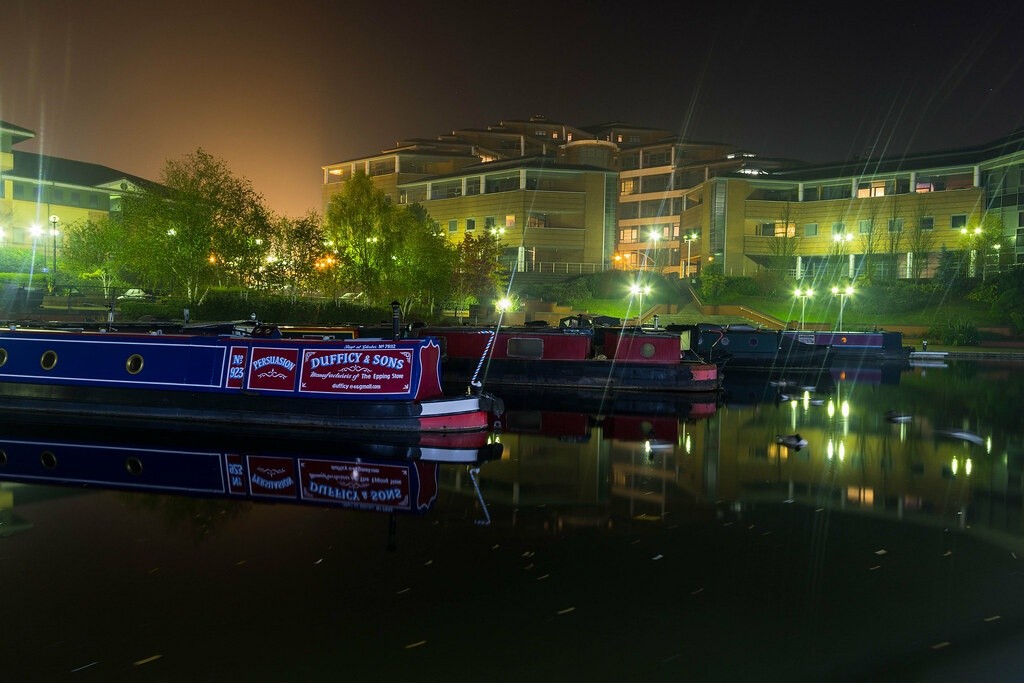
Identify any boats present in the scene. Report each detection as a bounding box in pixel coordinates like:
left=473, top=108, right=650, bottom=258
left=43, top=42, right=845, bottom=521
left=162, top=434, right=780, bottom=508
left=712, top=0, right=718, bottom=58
left=910, top=351, right=949, bottom=360
left=2, top=424, right=481, bottom=519
left=780, top=330, right=910, bottom=360
left=498, top=389, right=716, bottom=449
left=694, top=322, right=837, bottom=370
left=0, top=301, right=504, bottom=431
left=226, top=315, right=726, bottom=393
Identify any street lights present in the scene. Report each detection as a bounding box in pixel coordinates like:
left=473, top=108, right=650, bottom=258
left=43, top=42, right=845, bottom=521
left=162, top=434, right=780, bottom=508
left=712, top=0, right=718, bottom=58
left=491, top=229, right=504, bottom=274
left=832, top=287, right=853, bottom=332
left=630, top=286, right=650, bottom=323
left=50, top=215, right=59, bottom=272
left=795, top=290, right=813, bottom=330
left=682, top=234, right=697, bottom=276
